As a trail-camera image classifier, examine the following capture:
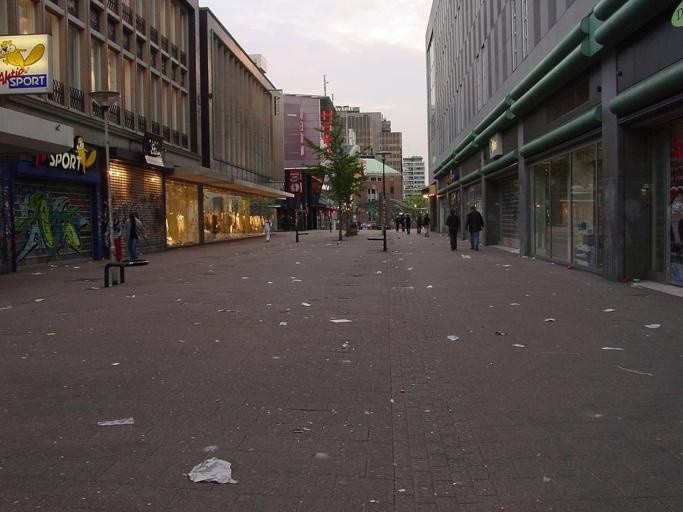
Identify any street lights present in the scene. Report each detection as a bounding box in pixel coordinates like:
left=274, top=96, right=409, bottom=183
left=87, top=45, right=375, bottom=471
left=375, top=151, right=392, bottom=252
left=88, top=90, right=121, bottom=287
left=289, top=177, right=300, bottom=242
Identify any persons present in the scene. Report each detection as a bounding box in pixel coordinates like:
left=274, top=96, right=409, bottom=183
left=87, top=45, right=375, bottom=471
left=262, top=218, right=272, bottom=242
left=465, top=206, right=484, bottom=251
left=400, top=214, right=405, bottom=231
left=395, top=218, right=400, bottom=231
left=405, top=214, right=410, bottom=234
left=423, top=214, right=429, bottom=237
left=123, top=214, right=144, bottom=260
left=416, top=216, right=421, bottom=233
left=445, top=209, right=458, bottom=251
